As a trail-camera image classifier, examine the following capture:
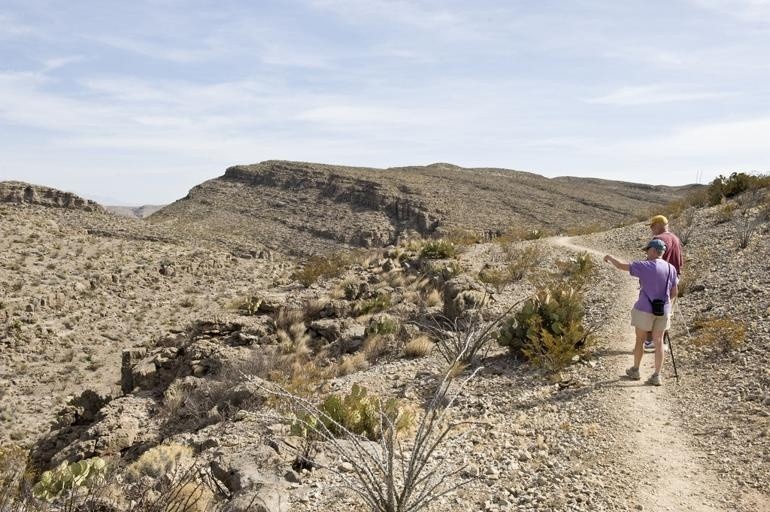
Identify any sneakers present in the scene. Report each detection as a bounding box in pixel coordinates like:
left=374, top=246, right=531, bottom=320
left=648, top=374, right=662, bottom=386
left=626, top=367, right=641, bottom=380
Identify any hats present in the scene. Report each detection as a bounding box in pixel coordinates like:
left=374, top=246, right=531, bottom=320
left=641, top=239, right=667, bottom=251
left=645, top=215, right=669, bottom=226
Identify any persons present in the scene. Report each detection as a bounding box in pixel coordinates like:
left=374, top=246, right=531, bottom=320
left=642, top=215, right=683, bottom=353
left=602, top=239, right=678, bottom=386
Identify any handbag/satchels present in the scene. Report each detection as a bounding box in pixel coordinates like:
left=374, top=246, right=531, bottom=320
left=652, top=299, right=665, bottom=316
